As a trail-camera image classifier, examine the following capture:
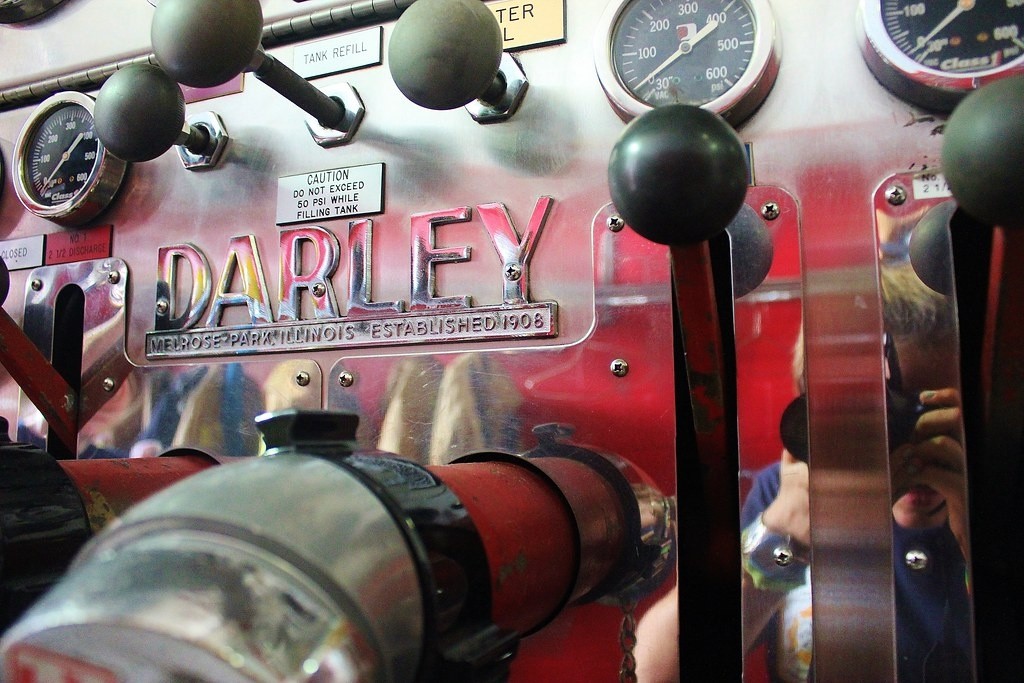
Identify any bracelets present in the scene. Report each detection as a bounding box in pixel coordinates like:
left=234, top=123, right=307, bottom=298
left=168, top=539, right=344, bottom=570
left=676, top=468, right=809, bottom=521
left=742, top=512, right=810, bottom=595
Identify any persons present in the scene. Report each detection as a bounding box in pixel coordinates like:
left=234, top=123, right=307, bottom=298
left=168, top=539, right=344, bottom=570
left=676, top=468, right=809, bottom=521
left=632, top=262, right=973, bottom=682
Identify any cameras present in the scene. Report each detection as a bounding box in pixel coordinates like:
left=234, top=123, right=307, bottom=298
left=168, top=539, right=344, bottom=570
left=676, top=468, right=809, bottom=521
left=779, top=373, right=935, bottom=461
